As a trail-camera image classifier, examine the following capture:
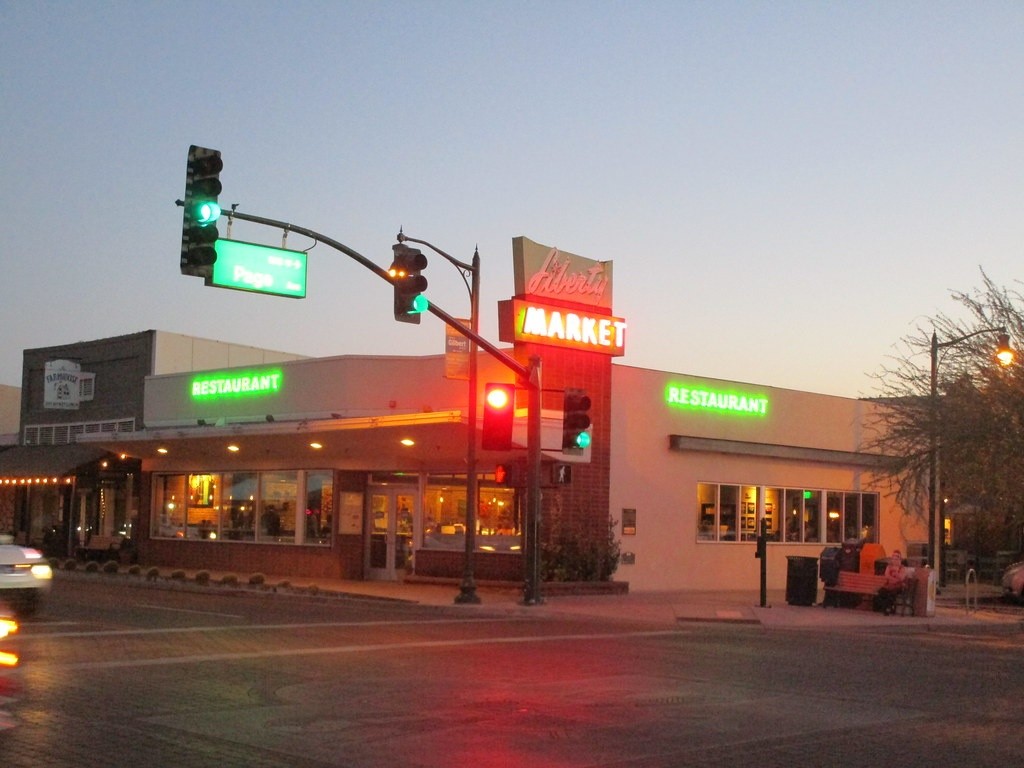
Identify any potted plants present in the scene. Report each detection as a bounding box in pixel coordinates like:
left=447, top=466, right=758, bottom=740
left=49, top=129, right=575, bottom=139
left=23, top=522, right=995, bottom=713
left=539, top=510, right=630, bottom=596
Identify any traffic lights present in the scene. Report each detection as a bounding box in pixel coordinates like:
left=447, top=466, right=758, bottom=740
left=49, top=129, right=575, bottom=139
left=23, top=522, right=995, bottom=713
left=482, top=383, right=515, bottom=451
left=562, top=391, right=592, bottom=456
left=495, top=463, right=512, bottom=486
left=551, top=463, right=571, bottom=484
left=180, top=145, right=223, bottom=278
left=394, top=248, right=429, bottom=324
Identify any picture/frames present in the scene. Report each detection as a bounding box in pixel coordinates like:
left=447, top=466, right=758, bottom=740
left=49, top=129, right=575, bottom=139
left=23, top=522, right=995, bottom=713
left=188, top=473, right=214, bottom=508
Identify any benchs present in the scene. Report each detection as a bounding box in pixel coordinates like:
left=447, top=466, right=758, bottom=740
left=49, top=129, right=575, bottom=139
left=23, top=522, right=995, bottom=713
left=823, top=571, right=918, bottom=616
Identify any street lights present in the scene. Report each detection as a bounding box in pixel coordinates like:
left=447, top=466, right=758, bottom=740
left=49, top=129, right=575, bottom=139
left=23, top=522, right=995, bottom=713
left=928, top=326, right=1014, bottom=568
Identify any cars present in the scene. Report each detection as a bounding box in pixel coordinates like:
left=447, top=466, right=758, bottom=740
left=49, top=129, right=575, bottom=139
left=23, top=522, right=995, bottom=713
left=0, top=544, right=53, bottom=614
left=1001, top=562, right=1024, bottom=606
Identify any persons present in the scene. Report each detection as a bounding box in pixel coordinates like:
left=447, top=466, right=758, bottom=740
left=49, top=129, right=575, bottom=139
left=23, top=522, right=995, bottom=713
left=875, top=549, right=906, bottom=617
left=228, top=502, right=333, bottom=537
left=784, top=514, right=875, bottom=545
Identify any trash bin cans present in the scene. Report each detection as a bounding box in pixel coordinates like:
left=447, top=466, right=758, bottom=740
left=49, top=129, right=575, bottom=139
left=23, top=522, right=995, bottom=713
left=783, top=555, right=819, bottom=605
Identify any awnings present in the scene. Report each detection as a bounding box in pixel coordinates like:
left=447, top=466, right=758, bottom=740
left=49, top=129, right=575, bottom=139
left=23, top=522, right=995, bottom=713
left=1, top=442, right=111, bottom=478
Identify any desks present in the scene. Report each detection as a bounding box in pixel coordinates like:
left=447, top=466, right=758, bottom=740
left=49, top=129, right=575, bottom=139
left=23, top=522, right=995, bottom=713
left=372, top=532, right=412, bottom=569
